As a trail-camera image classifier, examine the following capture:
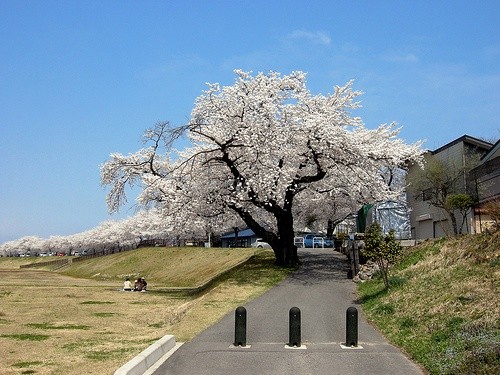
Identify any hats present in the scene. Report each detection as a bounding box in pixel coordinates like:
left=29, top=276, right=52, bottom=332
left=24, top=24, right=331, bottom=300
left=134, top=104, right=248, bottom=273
left=135, top=280, right=138, bottom=283
left=141, top=277, right=145, bottom=280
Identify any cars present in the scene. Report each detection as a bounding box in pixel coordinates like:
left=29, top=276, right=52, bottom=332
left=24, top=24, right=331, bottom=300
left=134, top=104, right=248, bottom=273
left=305, top=234, right=334, bottom=247
left=255, top=239, right=270, bottom=248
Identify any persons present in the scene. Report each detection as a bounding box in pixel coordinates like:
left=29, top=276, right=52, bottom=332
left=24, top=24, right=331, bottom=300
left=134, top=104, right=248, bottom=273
left=123, top=278, right=133, bottom=292
left=132, top=277, right=147, bottom=292
